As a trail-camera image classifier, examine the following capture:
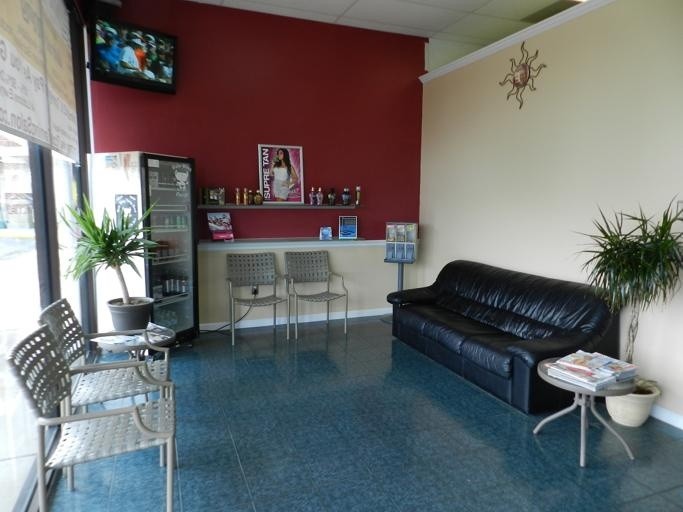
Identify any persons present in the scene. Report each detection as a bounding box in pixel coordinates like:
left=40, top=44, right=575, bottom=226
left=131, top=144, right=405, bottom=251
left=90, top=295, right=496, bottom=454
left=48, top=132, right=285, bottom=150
left=268, top=148, right=298, bottom=202
left=95, top=19, right=174, bottom=85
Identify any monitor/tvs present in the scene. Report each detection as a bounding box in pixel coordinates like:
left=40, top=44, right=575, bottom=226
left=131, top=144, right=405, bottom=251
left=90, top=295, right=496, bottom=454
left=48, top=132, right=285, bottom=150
left=89, top=15, right=178, bottom=95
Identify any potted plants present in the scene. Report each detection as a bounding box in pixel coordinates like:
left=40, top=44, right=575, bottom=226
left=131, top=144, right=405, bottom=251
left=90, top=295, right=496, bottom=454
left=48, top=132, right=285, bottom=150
left=58, top=193, right=160, bottom=335
left=574, top=192, right=683, bottom=428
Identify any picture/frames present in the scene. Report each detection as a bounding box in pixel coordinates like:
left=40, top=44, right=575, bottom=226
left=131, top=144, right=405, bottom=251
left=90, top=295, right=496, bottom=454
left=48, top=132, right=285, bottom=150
left=339, top=216, right=357, bottom=240
left=258, top=144, right=304, bottom=206
left=320, top=227, right=332, bottom=241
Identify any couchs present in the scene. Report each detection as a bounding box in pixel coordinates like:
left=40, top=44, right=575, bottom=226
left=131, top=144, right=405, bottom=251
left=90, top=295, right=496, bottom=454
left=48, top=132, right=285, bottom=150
left=387, top=260, right=627, bottom=415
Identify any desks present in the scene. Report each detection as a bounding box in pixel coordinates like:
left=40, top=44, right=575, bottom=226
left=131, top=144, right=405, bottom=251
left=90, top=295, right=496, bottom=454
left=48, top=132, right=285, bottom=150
left=91, top=324, right=176, bottom=361
left=533, top=356, right=637, bottom=468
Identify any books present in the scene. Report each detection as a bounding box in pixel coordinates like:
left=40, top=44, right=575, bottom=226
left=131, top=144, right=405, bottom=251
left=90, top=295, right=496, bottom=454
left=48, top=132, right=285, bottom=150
left=544, top=349, right=639, bottom=393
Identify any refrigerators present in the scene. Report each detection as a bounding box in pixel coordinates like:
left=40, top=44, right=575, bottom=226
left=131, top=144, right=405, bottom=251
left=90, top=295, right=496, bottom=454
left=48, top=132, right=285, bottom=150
left=88, top=152, right=199, bottom=346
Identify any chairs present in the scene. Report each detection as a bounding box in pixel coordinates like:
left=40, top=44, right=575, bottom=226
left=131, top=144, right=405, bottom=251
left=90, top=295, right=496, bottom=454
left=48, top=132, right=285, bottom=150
left=284, top=251, right=348, bottom=340
left=226, top=252, right=290, bottom=346
left=7, top=324, right=178, bottom=512
left=38, top=298, right=170, bottom=479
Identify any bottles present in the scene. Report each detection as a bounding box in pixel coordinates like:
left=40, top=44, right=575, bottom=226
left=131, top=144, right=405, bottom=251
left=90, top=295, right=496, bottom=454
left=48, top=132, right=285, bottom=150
left=234, top=188, right=241, bottom=206
left=316, top=187, right=324, bottom=206
left=354, top=184, right=361, bottom=205
left=150, top=270, right=162, bottom=303
left=243, top=188, right=249, bottom=206
left=253, top=191, right=263, bottom=205
left=327, top=187, right=337, bottom=206
left=308, top=187, right=316, bottom=206
left=163, top=270, right=169, bottom=296
left=174, top=269, right=181, bottom=294
left=169, top=270, right=176, bottom=295
left=150, top=239, right=188, bottom=262
left=180, top=271, right=187, bottom=293
left=248, top=190, right=254, bottom=205
left=341, top=185, right=351, bottom=206
